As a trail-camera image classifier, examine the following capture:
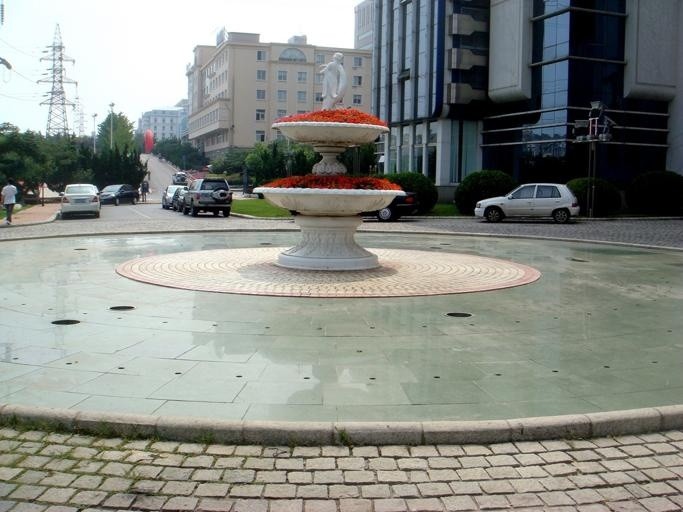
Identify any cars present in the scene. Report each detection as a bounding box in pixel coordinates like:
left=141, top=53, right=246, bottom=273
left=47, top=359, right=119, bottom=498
left=359, top=191, right=417, bottom=220
left=60, top=184, right=101, bottom=217
left=161, top=170, right=191, bottom=212
left=100, top=184, right=140, bottom=205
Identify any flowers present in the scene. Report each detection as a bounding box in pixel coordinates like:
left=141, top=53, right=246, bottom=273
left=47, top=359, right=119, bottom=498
left=273, top=108, right=387, bottom=127
left=262, top=174, right=402, bottom=190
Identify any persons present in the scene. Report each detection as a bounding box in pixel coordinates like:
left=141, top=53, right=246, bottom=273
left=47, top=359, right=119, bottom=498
left=140, top=181, right=148, bottom=202
left=0, top=177, right=17, bottom=226
left=317, top=52, right=344, bottom=99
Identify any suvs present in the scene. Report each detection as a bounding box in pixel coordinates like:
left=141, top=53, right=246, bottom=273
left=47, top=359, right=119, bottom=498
left=475, top=182, right=580, bottom=223
left=183, top=178, right=231, bottom=218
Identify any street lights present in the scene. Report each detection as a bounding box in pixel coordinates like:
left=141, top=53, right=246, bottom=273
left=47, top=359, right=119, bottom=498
left=90, top=102, right=114, bottom=157
